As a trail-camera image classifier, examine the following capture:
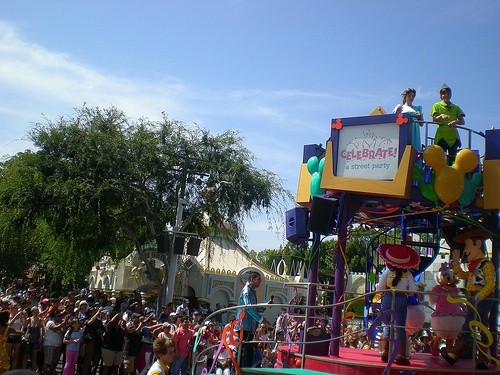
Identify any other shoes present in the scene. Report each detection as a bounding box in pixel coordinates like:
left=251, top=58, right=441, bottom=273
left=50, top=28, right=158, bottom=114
left=476, top=361, right=488, bottom=370
left=440, top=348, right=455, bottom=366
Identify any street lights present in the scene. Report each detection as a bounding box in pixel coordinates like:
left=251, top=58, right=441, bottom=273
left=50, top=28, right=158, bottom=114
left=157, top=149, right=210, bottom=305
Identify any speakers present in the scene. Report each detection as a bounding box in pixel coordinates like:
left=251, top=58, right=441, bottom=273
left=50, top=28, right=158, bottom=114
left=158, top=234, right=170, bottom=254
left=173, top=236, right=185, bottom=255
left=187, top=237, right=202, bottom=256
left=286, top=207, right=310, bottom=245
left=307, top=195, right=339, bottom=236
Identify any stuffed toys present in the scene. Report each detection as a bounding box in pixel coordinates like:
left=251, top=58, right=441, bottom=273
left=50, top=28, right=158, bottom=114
left=373, top=244, right=421, bottom=365
left=427, top=263, right=467, bottom=356
left=406, top=274, right=427, bottom=358
left=441, top=228, right=496, bottom=370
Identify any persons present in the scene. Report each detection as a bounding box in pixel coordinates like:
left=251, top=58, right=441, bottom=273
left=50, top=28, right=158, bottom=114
left=252, top=310, right=305, bottom=368
left=430, top=83, right=465, bottom=164
left=234, top=272, right=273, bottom=368
left=393, top=87, right=423, bottom=150
left=411, top=330, right=435, bottom=353
left=0, top=283, right=237, bottom=375
left=316, top=319, right=372, bottom=349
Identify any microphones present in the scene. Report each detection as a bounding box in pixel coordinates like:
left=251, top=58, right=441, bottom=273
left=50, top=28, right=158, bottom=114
left=270, top=296, right=274, bottom=309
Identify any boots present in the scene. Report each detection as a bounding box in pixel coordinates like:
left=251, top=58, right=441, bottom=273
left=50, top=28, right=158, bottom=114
left=381, top=338, right=389, bottom=362
left=397, top=338, right=411, bottom=365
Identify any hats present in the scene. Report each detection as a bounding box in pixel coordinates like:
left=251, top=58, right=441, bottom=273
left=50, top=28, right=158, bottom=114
left=162, top=321, right=171, bottom=329
left=79, top=301, right=88, bottom=307
left=184, top=299, right=189, bottom=303
left=442, top=83, right=450, bottom=89
left=378, top=244, right=421, bottom=269
